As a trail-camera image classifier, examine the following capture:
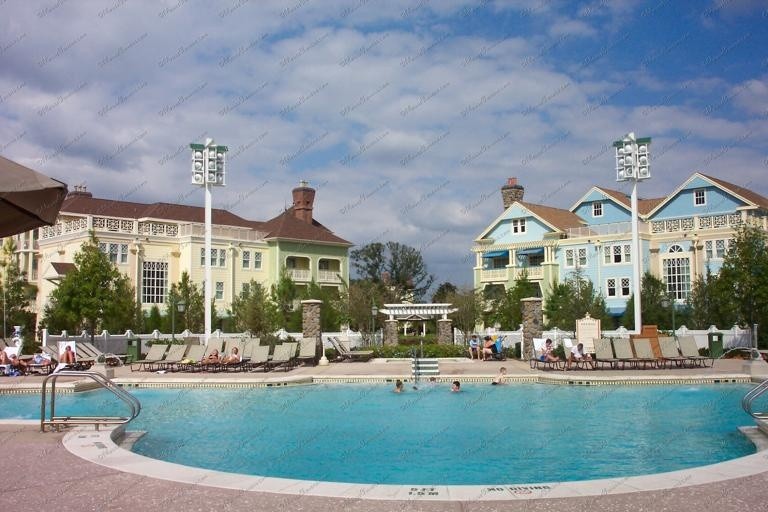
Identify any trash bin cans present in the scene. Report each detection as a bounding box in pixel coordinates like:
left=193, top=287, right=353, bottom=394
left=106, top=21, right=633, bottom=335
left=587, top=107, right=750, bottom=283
left=707, top=332, right=724, bottom=357
left=127, top=336, right=141, bottom=363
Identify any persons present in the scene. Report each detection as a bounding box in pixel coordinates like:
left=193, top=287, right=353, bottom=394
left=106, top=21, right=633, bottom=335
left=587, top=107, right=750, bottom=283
left=0, top=344, right=76, bottom=366
left=570, top=343, right=589, bottom=363
left=536, top=339, right=558, bottom=363
left=480, top=336, right=497, bottom=360
left=202, top=349, right=220, bottom=364
left=491, top=367, right=506, bottom=386
left=221, top=347, right=240, bottom=365
left=467, top=335, right=480, bottom=360
left=391, top=377, right=461, bottom=394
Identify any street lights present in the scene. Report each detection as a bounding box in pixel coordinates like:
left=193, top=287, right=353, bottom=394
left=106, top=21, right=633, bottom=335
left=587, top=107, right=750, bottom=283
left=370, top=305, right=378, bottom=332
left=188, top=137, right=227, bottom=345
left=612, top=131, right=652, bottom=337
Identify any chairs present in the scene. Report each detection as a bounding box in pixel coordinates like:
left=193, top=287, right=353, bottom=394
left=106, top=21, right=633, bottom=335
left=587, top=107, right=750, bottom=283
left=466, top=336, right=718, bottom=370
left=0, top=336, right=134, bottom=374
left=131, top=336, right=316, bottom=373
left=333, top=336, right=374, bottom=359
left=328, top=338, right=374, bottom=362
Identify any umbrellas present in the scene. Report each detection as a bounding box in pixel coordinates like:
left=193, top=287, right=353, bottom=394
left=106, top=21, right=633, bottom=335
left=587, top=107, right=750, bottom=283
left=0, top=154, right=68, bottom=238
